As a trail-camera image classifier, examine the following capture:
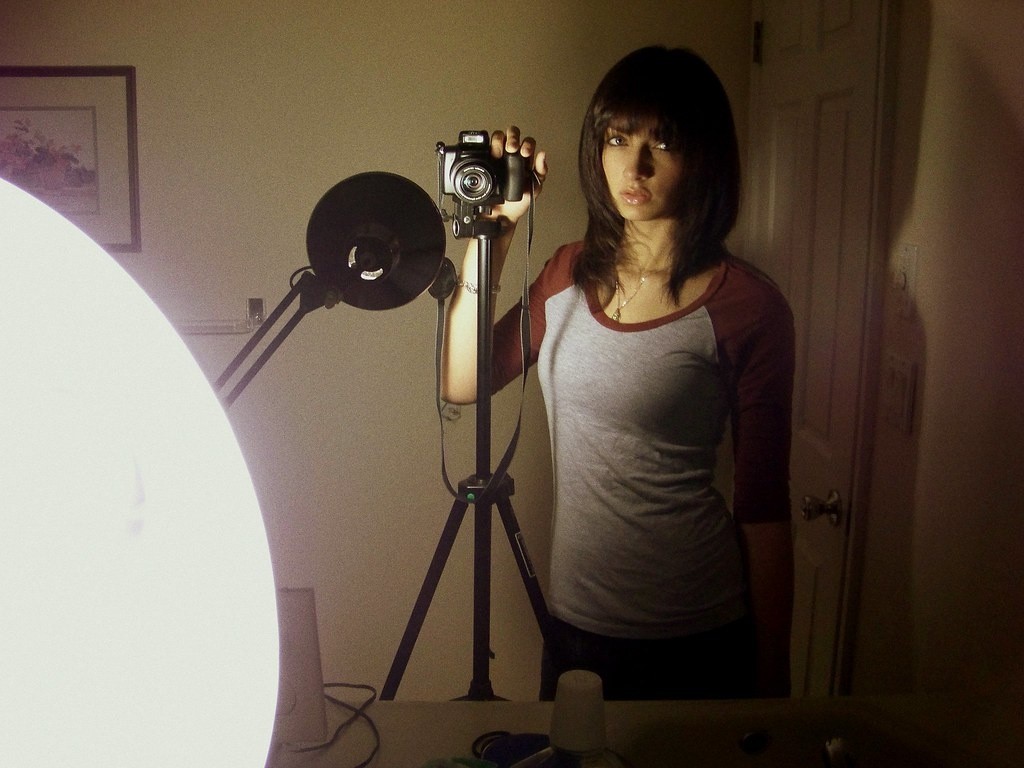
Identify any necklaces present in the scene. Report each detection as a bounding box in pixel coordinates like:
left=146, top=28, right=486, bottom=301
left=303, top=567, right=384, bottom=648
left=611, top=270, right=663, bottom=321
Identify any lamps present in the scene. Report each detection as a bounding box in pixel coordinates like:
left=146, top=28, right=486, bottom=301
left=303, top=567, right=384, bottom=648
left=210, top=170, right=458, bottom=415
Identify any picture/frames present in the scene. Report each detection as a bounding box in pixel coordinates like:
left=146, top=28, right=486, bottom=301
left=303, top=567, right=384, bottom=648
left=0, top=65, right=143, bottom=253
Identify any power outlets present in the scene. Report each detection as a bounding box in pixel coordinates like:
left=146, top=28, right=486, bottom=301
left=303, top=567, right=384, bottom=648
left=890, top=244, right=918, bottom=322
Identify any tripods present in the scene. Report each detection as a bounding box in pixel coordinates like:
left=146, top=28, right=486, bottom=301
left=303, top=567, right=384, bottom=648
left=378, top=208, right=575, bottom=701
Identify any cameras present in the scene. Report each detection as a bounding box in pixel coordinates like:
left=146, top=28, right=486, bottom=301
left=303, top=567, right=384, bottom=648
left=437, top=129, right=525, bottom=208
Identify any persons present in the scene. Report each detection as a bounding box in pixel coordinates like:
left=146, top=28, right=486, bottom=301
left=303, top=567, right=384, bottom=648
left=437, top=45, right=796, bottom=701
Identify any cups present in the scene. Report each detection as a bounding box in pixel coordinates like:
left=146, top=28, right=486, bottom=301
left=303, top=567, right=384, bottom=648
left=548, top=670, right=607, bottom=756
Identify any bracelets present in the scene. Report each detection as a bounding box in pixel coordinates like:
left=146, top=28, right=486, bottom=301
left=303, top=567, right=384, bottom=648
left=455, top=278, right=501, bottom=294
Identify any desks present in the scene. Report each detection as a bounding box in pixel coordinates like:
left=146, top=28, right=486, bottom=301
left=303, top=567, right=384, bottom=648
left=335, top=698, right=999, bottom=768
